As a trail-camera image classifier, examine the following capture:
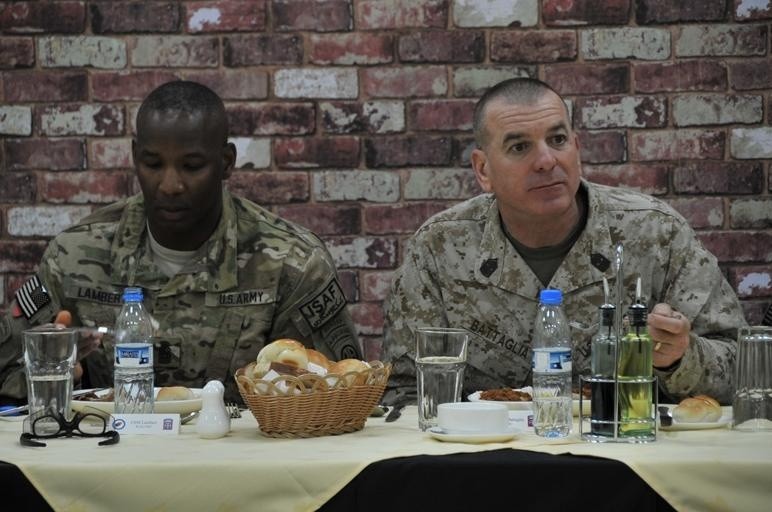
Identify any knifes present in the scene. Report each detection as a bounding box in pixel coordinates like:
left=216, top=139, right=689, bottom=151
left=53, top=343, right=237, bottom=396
left=385, top=403, right=405, bottom=422
left=658, top=406, right=672, bottom=427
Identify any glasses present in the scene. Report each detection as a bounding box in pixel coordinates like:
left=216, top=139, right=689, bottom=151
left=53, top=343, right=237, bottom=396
left=20, top=406, right=119, bottom=446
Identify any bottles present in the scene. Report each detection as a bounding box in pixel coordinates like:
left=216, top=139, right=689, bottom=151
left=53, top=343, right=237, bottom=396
left=113, top=288, right=154, bottom=415
left=194, top=379, right=231, bottom=439
left=589, top=304, right=655, bottom=440
left=531, top=290, right=574, bottom=438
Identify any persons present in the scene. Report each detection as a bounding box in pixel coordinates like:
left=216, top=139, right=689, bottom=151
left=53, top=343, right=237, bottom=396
left=380, top=77, right=751, bottom=403
left=0, top=81, right=365, bottom=407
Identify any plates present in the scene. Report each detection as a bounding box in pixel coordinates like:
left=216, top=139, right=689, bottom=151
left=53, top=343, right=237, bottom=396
left=71, top=388, right=204, bottom=419
left=425, top=422, right=525, bottom=444
left=467, top=390, right=586, bottom=422
left=656, top=416, right=731, bottom=431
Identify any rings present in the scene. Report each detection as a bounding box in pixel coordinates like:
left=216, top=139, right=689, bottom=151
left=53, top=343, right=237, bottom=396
left=653, top=341, right=661, bottom=352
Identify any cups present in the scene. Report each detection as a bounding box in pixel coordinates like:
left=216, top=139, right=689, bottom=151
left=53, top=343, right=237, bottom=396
left=435, top=401, right=512, bottom=435
left=414, top=326, right=466, bottom=430
left=731, top=326, right=772, bottom=432
left=20, top=328, right=79, bottom=434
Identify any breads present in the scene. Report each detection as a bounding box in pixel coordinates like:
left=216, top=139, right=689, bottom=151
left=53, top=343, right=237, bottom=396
left=156, top=386, right=194, bottom=402
left=242, top=337, right=371, bottom=393
left=669, top=394, right=722, bottom=425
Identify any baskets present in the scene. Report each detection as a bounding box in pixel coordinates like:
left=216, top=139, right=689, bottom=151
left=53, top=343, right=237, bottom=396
left=234, top=360, right=392, bottom=438
